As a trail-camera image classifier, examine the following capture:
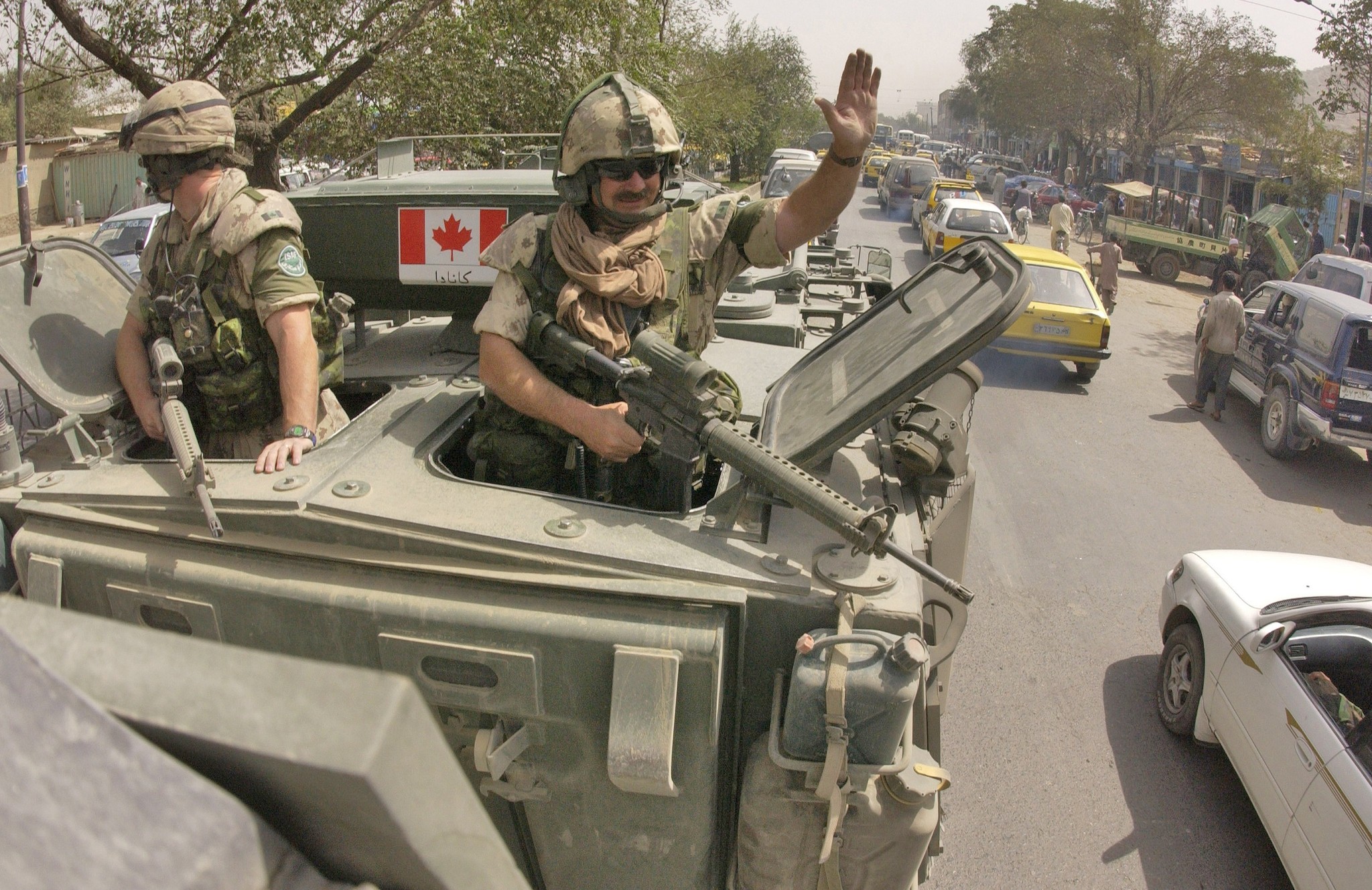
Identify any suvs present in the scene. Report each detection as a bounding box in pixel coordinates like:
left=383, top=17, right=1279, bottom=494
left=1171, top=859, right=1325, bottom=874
left=1192, top=279, right=1372, bottom=466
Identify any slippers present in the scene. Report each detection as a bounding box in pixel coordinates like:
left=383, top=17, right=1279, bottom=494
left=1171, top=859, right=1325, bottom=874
left=1187, top=402, right=1205, bottom=411
left=1210, top=411, right=1221, bottom=422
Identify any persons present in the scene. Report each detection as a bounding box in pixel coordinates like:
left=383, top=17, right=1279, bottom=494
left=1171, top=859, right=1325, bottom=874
left=1308, top=671, right=1365, bottom=736
left=977, top=211, right=997, bottom=227
left=1101, top=191, right=1237, bottom=242
left=1303, top=220, right=1370, bottom=262
left=132, top=176, right=148, bottom=209
left=1187, top=270, right=1246, bottom=420
left=1063, top=162, right=1080, bottom=189
left=1008, top=181, right=1033, bottom=232
left=990, top=166, right=1008, bottom=210
left=941, top=140, right=1057, bottom=179
left=467, top=47, right=882, bottom=496
left=1086, top=230, right=1123, bottom=316
left=1252, top=294, right=1296, bottom=328
left=1047, top=192, right=1075, bottom=256
left=901, top=143, right=917, bottom=156
left=113, top=79, right=320, bottom=473
left=1210, top=239, right=1244, bottom=297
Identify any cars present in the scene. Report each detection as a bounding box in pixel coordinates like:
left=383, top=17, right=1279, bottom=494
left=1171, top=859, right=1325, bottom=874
left=753, top=123, right=1096, bottom=260
left=974, top=241, right=1112, bottom=382
left=278, top=149, right=492, bottom=194
left=1155, top=550, right=1372, bottom=890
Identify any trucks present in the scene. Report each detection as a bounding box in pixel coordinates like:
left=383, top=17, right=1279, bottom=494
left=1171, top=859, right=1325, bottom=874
left=1100, top=183, right=1311, bottom=298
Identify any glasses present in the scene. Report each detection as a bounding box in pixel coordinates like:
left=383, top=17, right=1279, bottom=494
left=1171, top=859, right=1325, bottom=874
left=594, top=156, right=665, bottom=182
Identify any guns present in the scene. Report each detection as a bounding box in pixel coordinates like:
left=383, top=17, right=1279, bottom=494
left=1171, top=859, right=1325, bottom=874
left=528, top=313, right=979, bottom=607
left=147, top=333, right=228, bottom=543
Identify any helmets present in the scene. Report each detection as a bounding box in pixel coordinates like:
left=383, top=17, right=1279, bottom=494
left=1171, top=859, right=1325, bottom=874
left=118, top=80, right=236, bottom=156
left=558, top=72, right=682, bottom=176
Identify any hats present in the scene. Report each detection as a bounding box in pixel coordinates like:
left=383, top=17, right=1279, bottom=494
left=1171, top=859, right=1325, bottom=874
left=1360, top=232, right=1363, bottom=238
left=1229, top=239, right=1239, bottom=246
left=1338, top=235, right=1345, bottom=240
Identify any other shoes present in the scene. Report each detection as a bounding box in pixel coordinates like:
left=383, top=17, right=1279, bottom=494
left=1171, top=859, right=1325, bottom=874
left=1107, top=304, right=1114, bottom=315
left=1011, top=221, right=1017, bottom=227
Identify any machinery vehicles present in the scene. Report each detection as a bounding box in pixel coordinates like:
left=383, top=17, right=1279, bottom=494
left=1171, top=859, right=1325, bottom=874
left=0, top=124, right=1036, bottom=890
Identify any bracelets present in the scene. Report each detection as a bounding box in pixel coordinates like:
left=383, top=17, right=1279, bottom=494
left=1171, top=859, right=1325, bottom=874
left=829, top=142, right=864, bottom=167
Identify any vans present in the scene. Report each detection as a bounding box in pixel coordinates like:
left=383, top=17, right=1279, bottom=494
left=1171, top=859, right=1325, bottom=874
left=1286, top=252, right=1372, bottom=307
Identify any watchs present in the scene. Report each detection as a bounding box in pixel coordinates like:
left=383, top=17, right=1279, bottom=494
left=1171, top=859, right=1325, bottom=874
left=282, top=424, right=316, bottom=447
